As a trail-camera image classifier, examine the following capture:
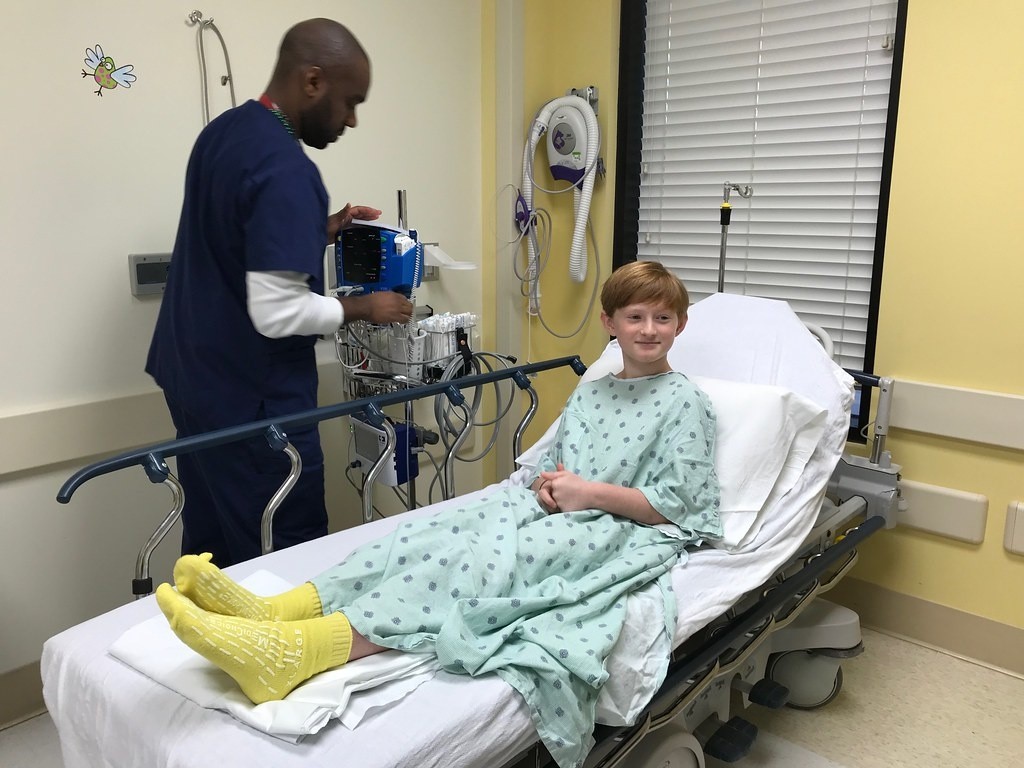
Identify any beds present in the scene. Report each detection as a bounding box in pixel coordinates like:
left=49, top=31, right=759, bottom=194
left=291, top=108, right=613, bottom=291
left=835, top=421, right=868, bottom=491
left=42, top=292, right=902, bottom=768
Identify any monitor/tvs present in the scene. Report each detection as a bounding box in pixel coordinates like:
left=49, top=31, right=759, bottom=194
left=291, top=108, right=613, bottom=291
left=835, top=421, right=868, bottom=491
left=335, top=227, right=424, bottom=294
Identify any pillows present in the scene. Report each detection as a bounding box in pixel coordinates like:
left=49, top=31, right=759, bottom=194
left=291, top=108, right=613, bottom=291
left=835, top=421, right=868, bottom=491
left=512, top=346, right=829, bottom=551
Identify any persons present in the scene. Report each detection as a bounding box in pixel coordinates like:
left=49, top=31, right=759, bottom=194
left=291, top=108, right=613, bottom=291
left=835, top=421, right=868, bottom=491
left=143, top=17, right=413, bottom=576
left=155, top=261, right=720, bottom=705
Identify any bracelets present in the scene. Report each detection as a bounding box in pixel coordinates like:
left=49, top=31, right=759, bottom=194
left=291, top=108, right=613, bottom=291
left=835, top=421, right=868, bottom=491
left=538, top=479, right=549, bottom=491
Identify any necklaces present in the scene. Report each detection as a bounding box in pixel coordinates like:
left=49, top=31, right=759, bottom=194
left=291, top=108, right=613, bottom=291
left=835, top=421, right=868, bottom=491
left=259, top=95, right=298, bottom=136
